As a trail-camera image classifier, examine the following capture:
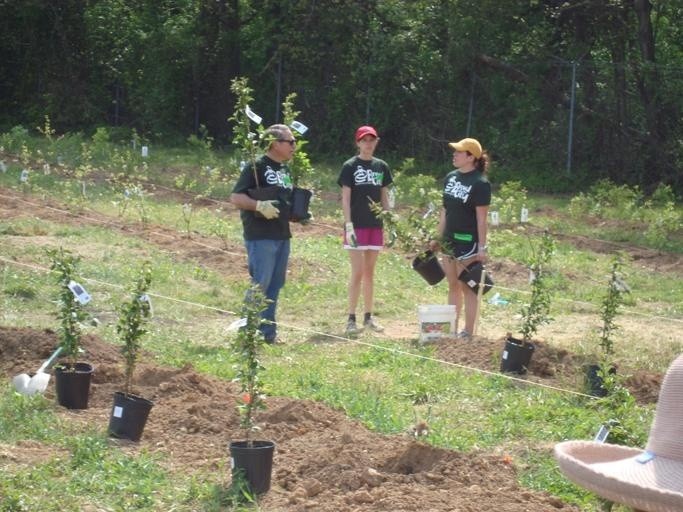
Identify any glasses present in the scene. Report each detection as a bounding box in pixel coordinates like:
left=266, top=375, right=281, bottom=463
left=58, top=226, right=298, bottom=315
left=277, top=138, right=297, bottom=146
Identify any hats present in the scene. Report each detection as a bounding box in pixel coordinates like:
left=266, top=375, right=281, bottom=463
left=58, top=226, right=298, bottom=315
left=447, top=137, right=483, bottom=160
left=354, top=125, right=378, bottom=143
left=557, top=351, right=683, bottom=512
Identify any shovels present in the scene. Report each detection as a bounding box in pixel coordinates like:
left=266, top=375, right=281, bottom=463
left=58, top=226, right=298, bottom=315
left=12, top=346, right=64, bottom=398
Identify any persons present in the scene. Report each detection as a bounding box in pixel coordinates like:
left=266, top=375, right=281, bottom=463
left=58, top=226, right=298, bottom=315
left=426, top=136, right=495, bottom=338
left=226, top=122, right=316, bottom=348
left=336, top=122, right=397, bottom=336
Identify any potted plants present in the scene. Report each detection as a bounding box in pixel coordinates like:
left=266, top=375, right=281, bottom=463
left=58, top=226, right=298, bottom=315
left=582, top=247, right=628, bottom=395
left=366, top=197, right=446, bottom=285
left=404, top=209, right=494, bottom=296
left=229, top=74, right=279, bottom=217
left=106, top=256, right=154, bottom=444
left=499, top=230, right=557, bottom=378
left=278, top=89, right=314, bottom=223
left=42, top=245, right=93, bottom=413
left=223, top=277, right=274, bottom=494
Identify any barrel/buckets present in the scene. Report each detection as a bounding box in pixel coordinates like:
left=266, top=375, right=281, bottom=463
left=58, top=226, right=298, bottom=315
left=417, top=304, right=458, bottom=343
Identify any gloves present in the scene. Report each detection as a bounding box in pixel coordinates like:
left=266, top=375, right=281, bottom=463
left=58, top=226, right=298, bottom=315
left=383, top=223, right=398, bottom=248
left=345, top=221, right=358, bottom=248
left=254, top=198, right=282, bottom=220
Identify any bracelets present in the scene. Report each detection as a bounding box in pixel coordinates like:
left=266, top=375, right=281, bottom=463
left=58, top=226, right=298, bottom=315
left=478, top=244, right=489, bottom=251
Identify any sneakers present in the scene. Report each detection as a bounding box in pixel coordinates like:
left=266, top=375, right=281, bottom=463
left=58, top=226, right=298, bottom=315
left=344, top=318, right=359, bottom=335
left=453, top=329, right=473, bottom=340
left=362, top=319, right=385, bottom=333
